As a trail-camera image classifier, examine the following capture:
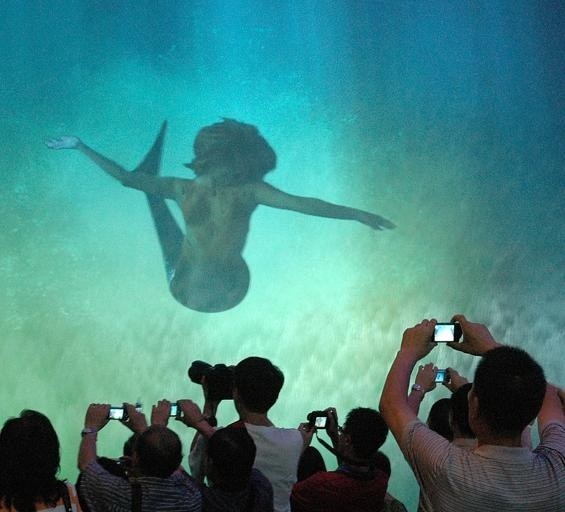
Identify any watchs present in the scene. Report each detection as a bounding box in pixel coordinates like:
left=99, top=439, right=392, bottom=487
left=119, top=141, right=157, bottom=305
left=204, top=416, right=217, bottom=427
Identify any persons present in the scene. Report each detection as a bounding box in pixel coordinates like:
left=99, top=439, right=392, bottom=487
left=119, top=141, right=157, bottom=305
left=97, top=432, right=136, bottom=479
left=43, top=116, right=398, bottom=312
left=0, top=410, right=84, bottom=512
left=151, top=399, right=273, bottom=512
left=188, top=356, right=303, bottom=512
left=77, top=401, right=203, bottom=512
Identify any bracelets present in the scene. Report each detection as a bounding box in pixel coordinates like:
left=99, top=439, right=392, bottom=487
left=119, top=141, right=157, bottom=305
left=81, top=428, right=97, bottom=438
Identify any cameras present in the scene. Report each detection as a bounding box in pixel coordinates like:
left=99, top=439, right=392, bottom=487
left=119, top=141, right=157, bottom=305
left=432, top=323, right=463, bottom=343
left=434, top=370, right=448, bottom=383
left=168, top=403, right=181, bottom=417
left=307, top=411, right=330, bottom=429
left=108, top=407, right=125, bottom=420
left=188, top=360, right=234, bottom=400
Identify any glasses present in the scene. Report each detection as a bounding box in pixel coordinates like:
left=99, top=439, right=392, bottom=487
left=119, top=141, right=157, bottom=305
left=338, top=426, right=346, bottom=436
